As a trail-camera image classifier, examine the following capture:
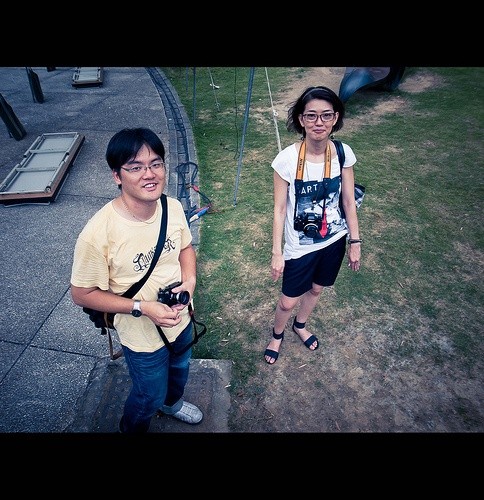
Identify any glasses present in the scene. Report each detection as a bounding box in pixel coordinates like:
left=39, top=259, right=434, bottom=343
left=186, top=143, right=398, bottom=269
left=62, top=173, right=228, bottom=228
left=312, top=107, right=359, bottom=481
left=302, top=113, right=336, bottom=123
left=120, top=163, right=168, bottom=176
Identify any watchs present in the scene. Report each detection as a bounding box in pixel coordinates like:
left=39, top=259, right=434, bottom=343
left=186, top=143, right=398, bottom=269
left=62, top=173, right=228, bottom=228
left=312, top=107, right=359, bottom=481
left=130, top=300, right=142, bottom=317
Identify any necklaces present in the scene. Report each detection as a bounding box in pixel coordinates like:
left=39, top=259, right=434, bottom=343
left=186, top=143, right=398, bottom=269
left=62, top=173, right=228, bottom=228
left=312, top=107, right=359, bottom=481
left=122, top=197, right=159, bottom=224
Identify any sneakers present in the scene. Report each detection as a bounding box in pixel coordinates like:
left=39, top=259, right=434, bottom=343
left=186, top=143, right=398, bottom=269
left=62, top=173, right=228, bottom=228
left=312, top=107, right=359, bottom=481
left=159, top=399, right=203, bottom=424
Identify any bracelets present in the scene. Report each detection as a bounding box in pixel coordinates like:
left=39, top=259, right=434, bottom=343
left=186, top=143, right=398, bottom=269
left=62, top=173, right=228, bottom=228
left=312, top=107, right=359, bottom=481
left=349, top=239, right=361, bottom=243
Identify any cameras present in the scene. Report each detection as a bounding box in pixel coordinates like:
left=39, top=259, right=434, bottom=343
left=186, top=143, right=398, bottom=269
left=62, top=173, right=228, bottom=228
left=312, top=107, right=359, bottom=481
left=294, top=213, right=322, bottom=238
left=157, top=281, right=191, bottom=308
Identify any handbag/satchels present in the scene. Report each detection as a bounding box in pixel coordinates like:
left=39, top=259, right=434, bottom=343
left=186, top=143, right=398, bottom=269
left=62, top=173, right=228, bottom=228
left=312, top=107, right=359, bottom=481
left=333, top=139, right=366, bottom=221
left=83, top=307, right=116, bottom=334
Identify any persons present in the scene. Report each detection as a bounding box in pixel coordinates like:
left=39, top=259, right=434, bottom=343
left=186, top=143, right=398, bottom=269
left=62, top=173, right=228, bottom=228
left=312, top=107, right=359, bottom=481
left=264, top=86, right=361, bottom=364
left=71, top=127, right=203, bottom=433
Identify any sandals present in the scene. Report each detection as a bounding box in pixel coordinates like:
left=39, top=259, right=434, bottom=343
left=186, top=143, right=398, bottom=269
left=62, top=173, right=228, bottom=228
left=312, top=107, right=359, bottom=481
left=264, top=327, right=284, bottom=365
left=292, top=316, right=320, bottom=350
